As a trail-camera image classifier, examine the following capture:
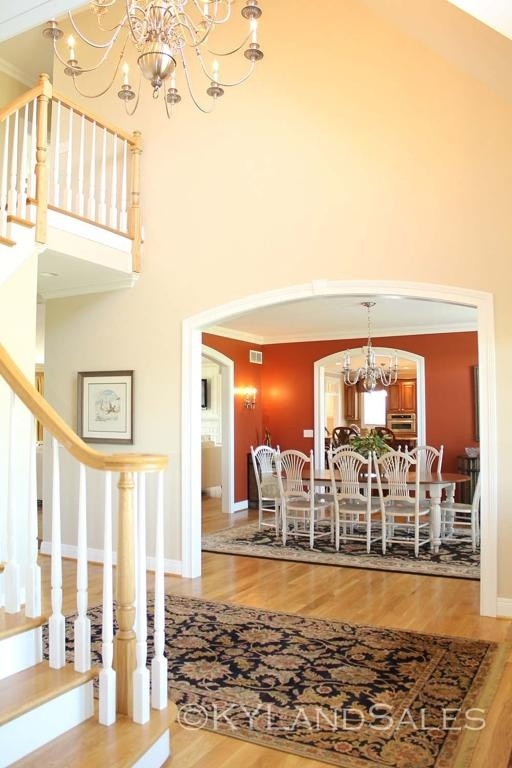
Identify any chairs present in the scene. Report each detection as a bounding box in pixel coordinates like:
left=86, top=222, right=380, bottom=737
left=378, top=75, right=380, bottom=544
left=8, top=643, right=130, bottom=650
left=249, top=425, right=480, bottom=557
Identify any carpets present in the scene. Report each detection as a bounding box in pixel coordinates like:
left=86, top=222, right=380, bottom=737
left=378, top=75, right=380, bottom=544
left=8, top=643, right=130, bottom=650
left=42, top=591, right=511, bottom=767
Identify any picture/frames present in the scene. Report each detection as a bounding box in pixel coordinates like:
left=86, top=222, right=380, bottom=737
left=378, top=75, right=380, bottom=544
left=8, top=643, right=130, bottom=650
left=77, top=370, right=135, bottom=446
left=202, top=515, right=481, bottom=580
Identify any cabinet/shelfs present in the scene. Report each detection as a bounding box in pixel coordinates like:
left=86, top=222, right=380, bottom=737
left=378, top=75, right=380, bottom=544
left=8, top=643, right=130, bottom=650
left=344, top=380, right=361, bottom=421
left=385, top=378, right=418, bottom=413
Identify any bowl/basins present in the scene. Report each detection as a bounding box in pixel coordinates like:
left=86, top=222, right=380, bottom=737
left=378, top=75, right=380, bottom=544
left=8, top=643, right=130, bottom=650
left=465, top=447, right=478, bottom=457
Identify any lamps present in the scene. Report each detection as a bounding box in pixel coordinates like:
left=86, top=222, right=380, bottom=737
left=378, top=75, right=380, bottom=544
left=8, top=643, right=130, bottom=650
left=41, top=0, right=264, bottom=120
left=342, top=301, right=399, bottom=393
left=242, top=385, right=257, bottom=411
